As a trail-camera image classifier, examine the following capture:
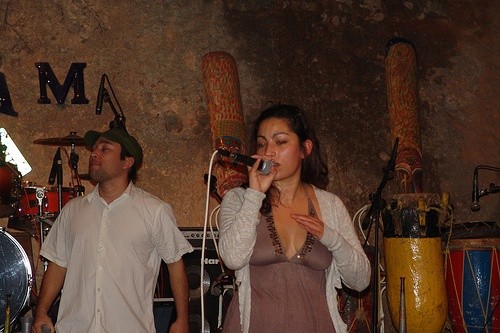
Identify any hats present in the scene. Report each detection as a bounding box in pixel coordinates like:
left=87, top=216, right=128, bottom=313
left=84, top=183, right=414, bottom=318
left=85, top=128, right=144, bottom=173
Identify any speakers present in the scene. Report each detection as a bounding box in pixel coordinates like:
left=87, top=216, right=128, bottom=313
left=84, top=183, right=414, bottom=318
left=156, top=226, right=236, bottom=333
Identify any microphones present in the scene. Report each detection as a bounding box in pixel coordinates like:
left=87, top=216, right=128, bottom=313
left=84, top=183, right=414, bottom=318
left=471, top=169, right=480, bottom=211
left=49, top=148, right=60, bottom=185
left=217, top=149, right=271, bottom=175
left=96, top=75, right=106, bottom=115
left=41, top=324, right=51, bottom=333
left=387, top=137, right=399, bottom=180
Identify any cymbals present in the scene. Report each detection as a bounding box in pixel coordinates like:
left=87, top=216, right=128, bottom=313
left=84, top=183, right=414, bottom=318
left=33, top=135, right=84, bottom=147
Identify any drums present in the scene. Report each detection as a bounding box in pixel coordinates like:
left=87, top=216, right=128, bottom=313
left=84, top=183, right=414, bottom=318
left=382, top=193, right=448, bottom=333
left=13, top=185, right=84, bottom=216
left=335, top=245, right=377, bottom=333
left=0, top=226, right=34, bottom=333
left=443, top=220, right=500, bottom=333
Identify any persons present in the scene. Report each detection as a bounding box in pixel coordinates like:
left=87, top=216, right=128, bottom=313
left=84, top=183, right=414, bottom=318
left=31, top=128, right=195, bottom=333
left=218, top=106, right=371, bottom=333
